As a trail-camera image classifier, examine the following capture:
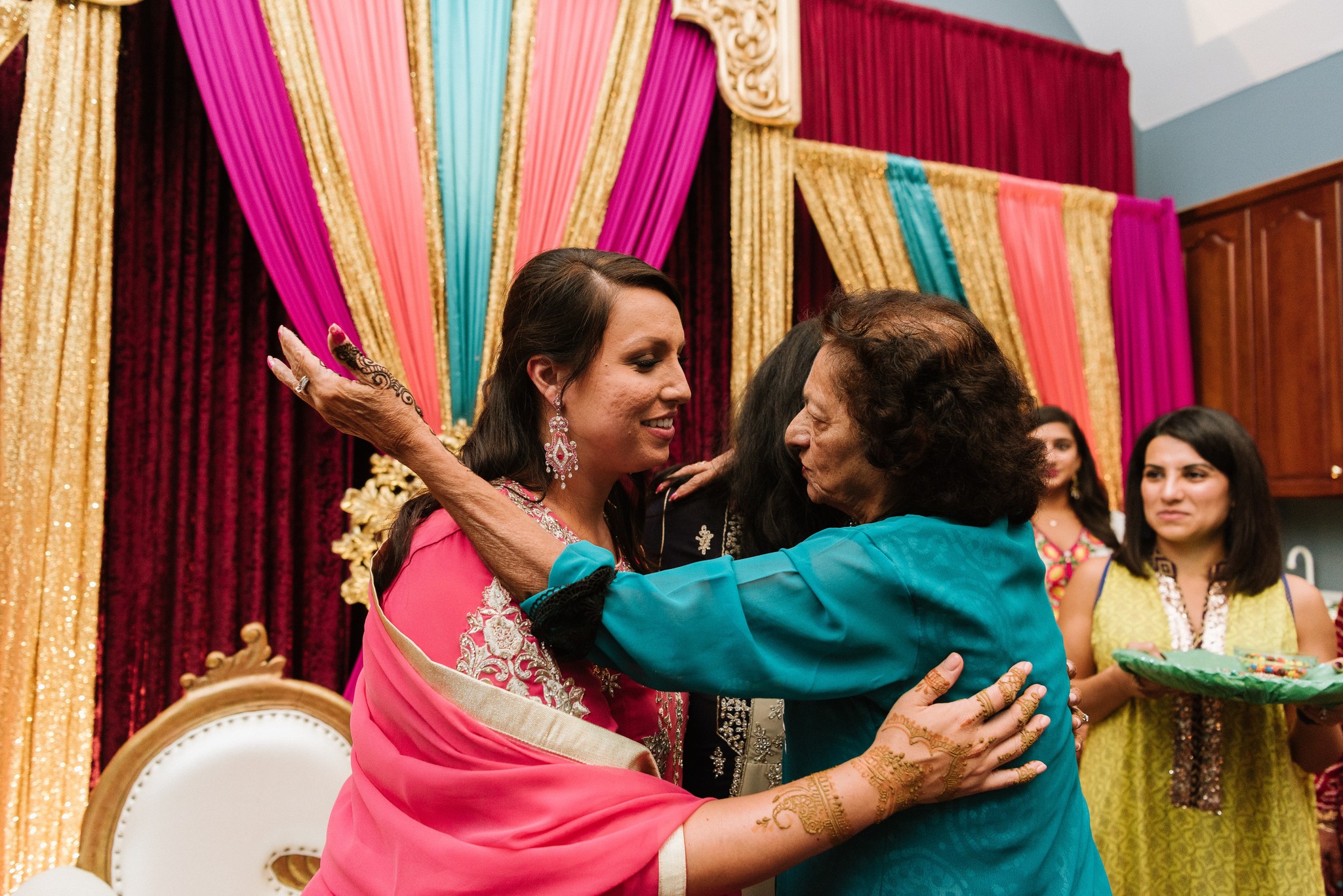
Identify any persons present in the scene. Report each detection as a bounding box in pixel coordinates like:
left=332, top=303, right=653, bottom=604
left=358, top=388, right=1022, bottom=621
left=1048, top=403, right=1343, bottom=896
left=1312, top=594, right=1343, bottom=895
left=603, top=314, right=843, bottom=896
left=264, top=246, right=1119, bottom=896
left=1019, top=401, right=1123, bottom=635
left=297, top=247, right=1051, bottom=896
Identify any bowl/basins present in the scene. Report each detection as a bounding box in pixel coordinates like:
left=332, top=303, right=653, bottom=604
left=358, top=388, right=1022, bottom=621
left=1237, top=650, right=1316, bottom=681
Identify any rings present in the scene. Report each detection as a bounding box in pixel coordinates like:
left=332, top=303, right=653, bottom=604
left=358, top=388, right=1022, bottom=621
left=294, top=375, right=310, bottom=397
left=1071, top=706, right=1089, bottom=723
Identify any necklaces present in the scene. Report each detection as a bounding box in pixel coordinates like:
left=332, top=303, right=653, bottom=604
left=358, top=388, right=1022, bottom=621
left=1049, top=519, right=1058, bottom=527
